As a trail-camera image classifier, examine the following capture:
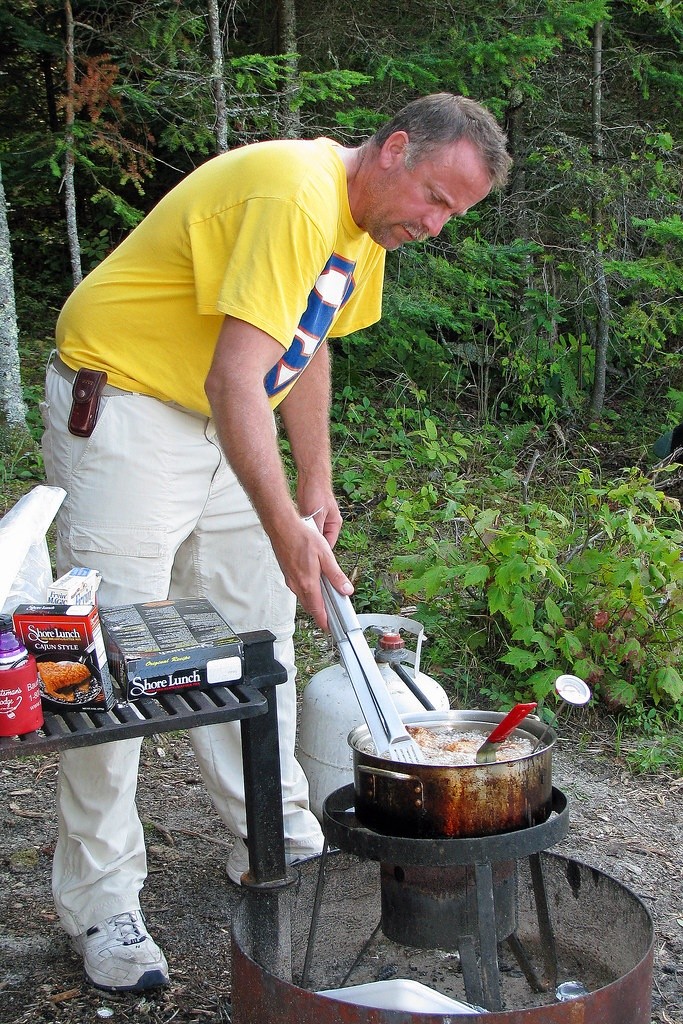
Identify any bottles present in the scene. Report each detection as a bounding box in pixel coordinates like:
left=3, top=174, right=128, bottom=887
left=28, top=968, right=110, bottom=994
left=0, top=614, right=43, bottom=670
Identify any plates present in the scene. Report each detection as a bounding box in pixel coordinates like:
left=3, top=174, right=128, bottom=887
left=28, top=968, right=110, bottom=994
left=34, top=652, right=103, bottom=706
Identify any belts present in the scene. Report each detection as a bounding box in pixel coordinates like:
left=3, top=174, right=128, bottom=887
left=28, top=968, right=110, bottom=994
left=52, top=355, right=133, bottom=396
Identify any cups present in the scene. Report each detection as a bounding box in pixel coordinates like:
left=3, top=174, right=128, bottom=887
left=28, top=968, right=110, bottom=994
left=0, top=653, right=44, bottom=737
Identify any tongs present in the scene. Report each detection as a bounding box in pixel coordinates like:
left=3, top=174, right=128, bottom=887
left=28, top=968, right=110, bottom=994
left=299, top=516, right=426, bottom=765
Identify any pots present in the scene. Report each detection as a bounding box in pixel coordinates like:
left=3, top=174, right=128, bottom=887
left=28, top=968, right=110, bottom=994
left=346, top=708, right=559, bottom=841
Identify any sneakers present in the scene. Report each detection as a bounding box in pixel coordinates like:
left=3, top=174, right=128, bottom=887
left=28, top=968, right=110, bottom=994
left=70, top=909, right=169, bottom=992
left=223, top=835, right=305, bottom=886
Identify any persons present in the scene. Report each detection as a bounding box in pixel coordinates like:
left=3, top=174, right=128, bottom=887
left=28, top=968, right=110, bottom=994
left=43, top=89, right=513, bottom=991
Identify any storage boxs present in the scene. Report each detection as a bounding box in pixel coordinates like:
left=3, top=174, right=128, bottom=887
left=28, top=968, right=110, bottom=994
left=14, top=605, right=117, bottom=714
left=100, top=595, right=246, bottom=704
left=50, top=565, right=108, bottom=610
